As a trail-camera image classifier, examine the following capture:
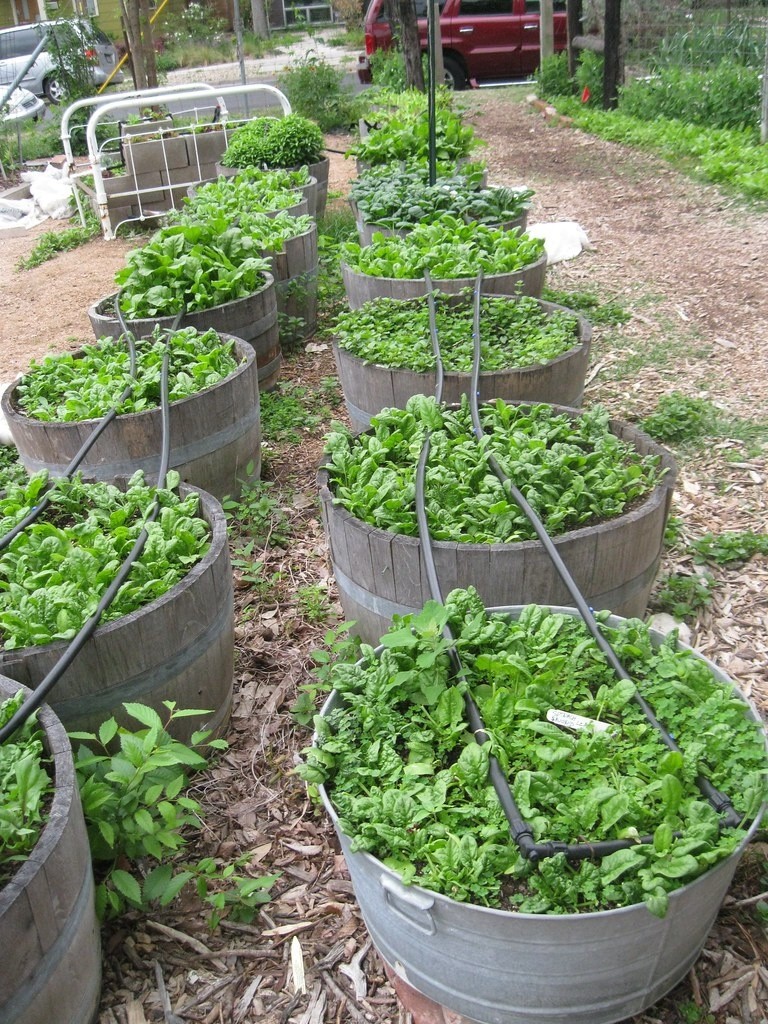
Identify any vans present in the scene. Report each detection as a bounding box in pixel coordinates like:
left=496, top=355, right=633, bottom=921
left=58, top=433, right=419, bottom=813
left=0, top=20, right=123, bottom=107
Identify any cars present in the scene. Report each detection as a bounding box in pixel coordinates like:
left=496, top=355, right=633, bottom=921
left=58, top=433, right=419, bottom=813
left=356, top=1, right=571, bottom=91
